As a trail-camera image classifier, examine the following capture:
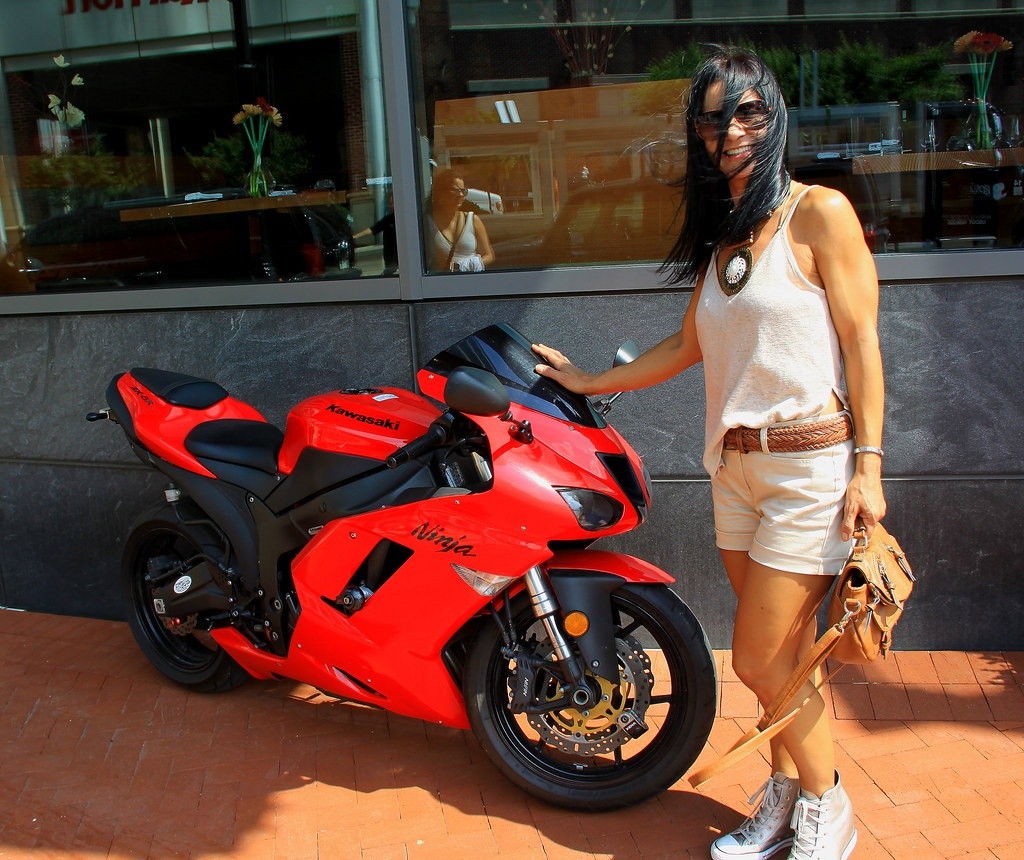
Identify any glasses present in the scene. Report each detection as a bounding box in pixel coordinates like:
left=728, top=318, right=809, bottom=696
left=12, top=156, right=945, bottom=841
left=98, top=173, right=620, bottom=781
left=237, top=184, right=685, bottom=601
left=694, top=100, right=767, bottom=141
left=446, top=189, right=468, bottom=196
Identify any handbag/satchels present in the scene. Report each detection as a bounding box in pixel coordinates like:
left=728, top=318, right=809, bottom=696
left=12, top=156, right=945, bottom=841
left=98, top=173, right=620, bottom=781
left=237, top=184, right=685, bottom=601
left=827, top=521, right=916, bottom=664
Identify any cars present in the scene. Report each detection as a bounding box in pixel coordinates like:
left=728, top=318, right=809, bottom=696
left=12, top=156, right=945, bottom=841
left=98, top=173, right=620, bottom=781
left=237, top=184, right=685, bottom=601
left=481, top=177, right=690, bottom=264
left=429, top=158, right=504, bottom=216
left=885, top=167, right=1024, bottom=256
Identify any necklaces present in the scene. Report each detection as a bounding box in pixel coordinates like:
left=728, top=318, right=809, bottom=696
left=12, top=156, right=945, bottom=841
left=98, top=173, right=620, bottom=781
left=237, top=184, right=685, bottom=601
left=719, top=230, right=754, bottom=295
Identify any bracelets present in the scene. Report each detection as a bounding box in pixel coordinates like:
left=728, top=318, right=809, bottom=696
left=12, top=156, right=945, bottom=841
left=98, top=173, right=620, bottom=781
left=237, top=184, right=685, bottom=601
left=854, top=447, right=884, bottom=457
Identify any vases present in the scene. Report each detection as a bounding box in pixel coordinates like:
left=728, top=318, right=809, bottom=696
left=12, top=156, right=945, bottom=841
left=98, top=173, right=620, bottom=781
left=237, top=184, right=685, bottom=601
left=965, top=102, right=1001, bottom=150
left=53, top=120, right=70, bottom=157
left=244, top=167, right=276, bottom=198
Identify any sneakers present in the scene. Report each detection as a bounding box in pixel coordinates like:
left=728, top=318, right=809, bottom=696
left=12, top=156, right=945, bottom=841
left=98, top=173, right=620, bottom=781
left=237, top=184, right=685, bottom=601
left=711, top=772, right=800, bottom=860
left=786, top=769, right=857, bottom=860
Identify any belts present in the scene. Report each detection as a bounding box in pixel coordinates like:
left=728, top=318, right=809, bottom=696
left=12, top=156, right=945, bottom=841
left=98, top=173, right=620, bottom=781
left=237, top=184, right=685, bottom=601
left=724, top=416, right=852, bottom=452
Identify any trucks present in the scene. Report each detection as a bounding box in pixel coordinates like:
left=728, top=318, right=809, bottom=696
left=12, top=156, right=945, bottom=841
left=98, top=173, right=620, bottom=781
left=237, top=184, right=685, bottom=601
left=780, top=97, right=1009, bottom=218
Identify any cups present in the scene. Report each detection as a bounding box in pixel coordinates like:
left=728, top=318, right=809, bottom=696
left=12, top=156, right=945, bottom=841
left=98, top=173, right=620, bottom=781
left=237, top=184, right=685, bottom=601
left=845, top=116, right=865, bottom=156
left=315, top=179, right=337, bottom=193
left=879, top=104, right=905, bottom=154
left=864, top=224, right=875, bottom=253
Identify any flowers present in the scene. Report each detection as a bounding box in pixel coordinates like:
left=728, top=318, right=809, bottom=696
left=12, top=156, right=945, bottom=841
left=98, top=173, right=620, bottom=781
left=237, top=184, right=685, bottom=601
left=954, top=30, right=1014, bottom=101
left=233, top=97, right=282, bottom=167
left=47, top=54, right=85, bottom=126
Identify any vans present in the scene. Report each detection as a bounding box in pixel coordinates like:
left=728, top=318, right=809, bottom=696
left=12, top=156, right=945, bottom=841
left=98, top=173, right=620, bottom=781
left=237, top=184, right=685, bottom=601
left=794, top=153, right=923, bottom=251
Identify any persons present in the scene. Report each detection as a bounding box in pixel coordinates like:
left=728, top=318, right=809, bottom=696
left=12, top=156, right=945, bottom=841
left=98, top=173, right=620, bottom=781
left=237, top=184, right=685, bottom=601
left=352, top=193, right=398, bottom=277
left=532, top=48, right=888, bottom=860
left=421, top=169, right=494, bottom=273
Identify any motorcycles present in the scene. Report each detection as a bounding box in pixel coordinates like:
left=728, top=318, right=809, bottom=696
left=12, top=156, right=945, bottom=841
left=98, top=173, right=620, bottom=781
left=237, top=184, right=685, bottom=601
left=85, top=325, right=716, bottom=814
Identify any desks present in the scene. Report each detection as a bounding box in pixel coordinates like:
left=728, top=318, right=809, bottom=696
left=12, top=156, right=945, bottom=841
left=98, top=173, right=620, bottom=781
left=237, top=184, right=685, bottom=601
left=119, top=188, right=347, bottom=284
left=852, top=148, right=1024, bottom=251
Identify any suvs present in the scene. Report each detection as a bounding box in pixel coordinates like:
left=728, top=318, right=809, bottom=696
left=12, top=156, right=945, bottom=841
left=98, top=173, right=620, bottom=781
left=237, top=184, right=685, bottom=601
left=0, top=179, right=365, bottom=283
